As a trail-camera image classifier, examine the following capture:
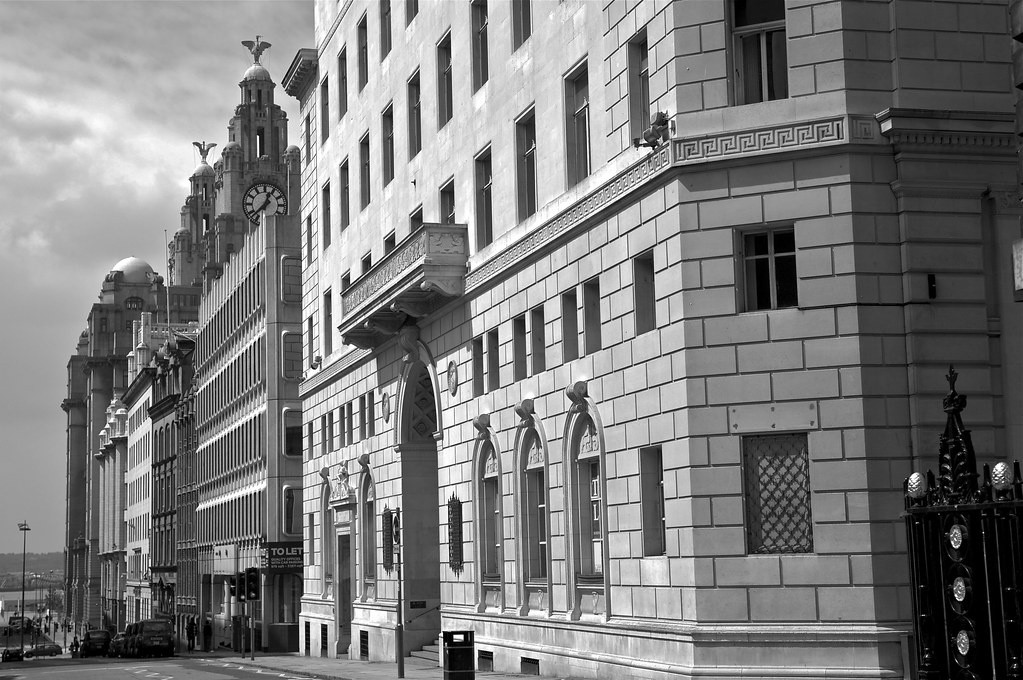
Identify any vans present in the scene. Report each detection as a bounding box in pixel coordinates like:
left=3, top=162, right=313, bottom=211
left=9, top=617, right=32, bottom=626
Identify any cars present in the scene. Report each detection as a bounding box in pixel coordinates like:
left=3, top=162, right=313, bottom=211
left=79, top=630, right=111, bottom=658
left=1, top=649, right=24, bottom=661
left=23, top=644, right=62, bottom=658
left=109, top=619, right=176, bottom=657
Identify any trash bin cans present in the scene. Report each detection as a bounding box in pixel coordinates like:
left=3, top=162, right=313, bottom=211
left=4, top=627, right=13, bottom=636
left=232, top=615, right=251, bottom=653
left=443, top=630, right=475, bottom=680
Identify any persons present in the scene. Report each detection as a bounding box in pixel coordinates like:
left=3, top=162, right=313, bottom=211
left=70, top=642, right=74, bottom=651
left=56, top=622, right=58, bottom=629
left=203, top=621, right=212, bottom=652
left=74, top=637, right=79, bottom=651
left=186, top=618, right=197, bottom=653
left=45, top=615, right=48, bottom=622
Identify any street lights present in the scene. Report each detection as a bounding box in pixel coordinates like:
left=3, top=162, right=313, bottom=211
left=18, top=520, right=31, bottom=652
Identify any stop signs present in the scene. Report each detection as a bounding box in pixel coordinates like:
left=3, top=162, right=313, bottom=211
left=394, top=516, right=400, bottom=542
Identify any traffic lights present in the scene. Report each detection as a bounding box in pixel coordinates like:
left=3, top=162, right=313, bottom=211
left=68, top=625, right=72, bottom=633
left=245, top=568, right=261, bottom=602
left=54, top=623, right=58, bottom=630
left=230, top=572, right=239, bottom=596
